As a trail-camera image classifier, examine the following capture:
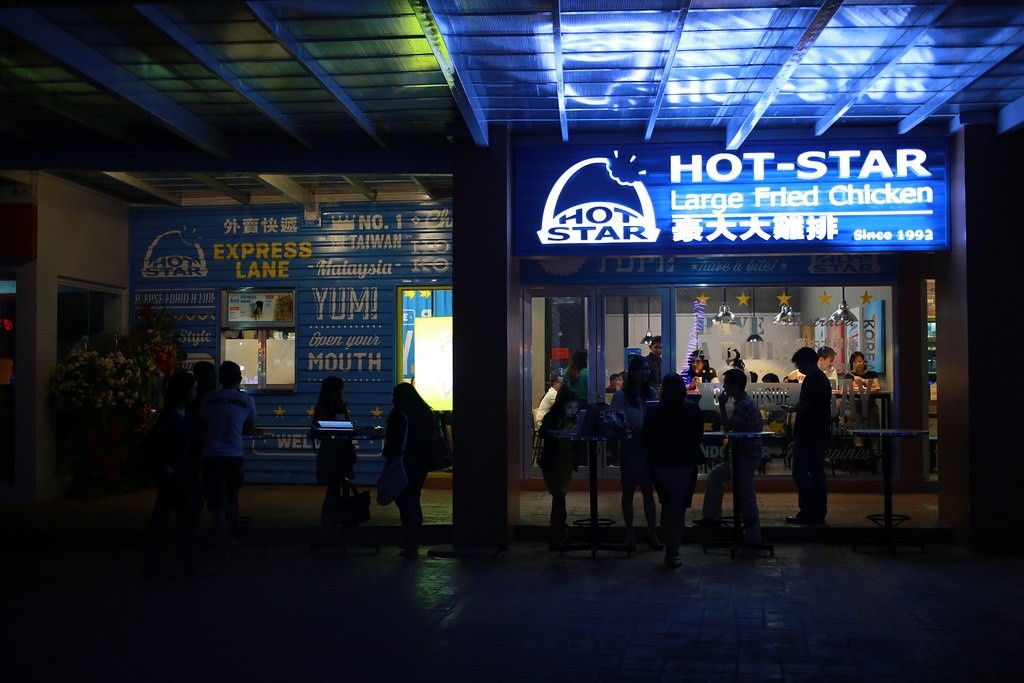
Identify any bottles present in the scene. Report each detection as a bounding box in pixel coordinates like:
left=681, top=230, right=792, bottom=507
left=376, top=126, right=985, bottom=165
left=928, top=351, right=936, bottom=373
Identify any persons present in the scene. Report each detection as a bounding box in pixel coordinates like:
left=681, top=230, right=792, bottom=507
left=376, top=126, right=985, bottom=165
left=140, top=360, right=257, bottom=582
left=610, top=356, right=664, bottom=551
left=782, top=347, right=831, bottom=525
left=844, top=351, right=881, bottom=449
left=692, top=368, right=765, bottom=542
left=786, top=346, right=839, bottom=392
left=537, top=391, right=586, bottom=549
left=310, top=375, right=358, bottom=546
left=640, top=371, right=704, bottom=560
left=536, top=376, right=562, bottom=430
left=608, top=337, right=797, bottom=435
left=562, top=349, right=588, bottom=409
left=380, top=383, right=448, bottom=557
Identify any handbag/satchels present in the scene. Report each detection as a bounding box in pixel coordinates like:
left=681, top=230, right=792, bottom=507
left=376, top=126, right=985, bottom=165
left=425, top=436, right=452, bottom=472
left=337, top=481, right=370, bottom=525
left=582, top=403, right=627, bottom=436
left=377, top=456, right=408, bottom=505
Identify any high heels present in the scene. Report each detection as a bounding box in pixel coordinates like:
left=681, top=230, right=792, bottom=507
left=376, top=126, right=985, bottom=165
left=647, top=533, right=665, bottom=550
left=628, top=538, right=637, bottom=551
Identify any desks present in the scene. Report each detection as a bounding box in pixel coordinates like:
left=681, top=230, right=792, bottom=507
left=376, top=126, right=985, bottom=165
left=313, top=425, right=386, bottom=555
left=686, top=391, right=893, bottom=479
left=554, top=431, right=636, bottom=561
left=846, top=428, right=930, bottom=558
left=702, top=431, right=774, bottom=559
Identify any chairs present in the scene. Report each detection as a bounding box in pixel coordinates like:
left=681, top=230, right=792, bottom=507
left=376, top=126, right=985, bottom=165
left=531, top=408, right=543, bottom=466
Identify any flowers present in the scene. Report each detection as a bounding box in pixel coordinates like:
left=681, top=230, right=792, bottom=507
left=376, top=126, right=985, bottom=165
left=48, top=301, right=189, bottom=436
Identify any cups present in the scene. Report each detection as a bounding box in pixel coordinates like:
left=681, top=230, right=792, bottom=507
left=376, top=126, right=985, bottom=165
left=695, top=377, right=702, bottom=392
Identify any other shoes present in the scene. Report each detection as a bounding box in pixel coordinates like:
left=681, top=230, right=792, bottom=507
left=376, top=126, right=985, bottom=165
left=399, top=547, right=417, bottom=558
left=548, top=540, right=566, bottom=551
left=186, top=567, right=218, bottom=580
left=664, top=553, right=681, bottom=563
left=693, top=516, right=721, bottom=528
left=397, top=535, right=420, bottom=549
left=560, top=530, right=569, bottom=546
left=785, top=511, right=825, bottom=523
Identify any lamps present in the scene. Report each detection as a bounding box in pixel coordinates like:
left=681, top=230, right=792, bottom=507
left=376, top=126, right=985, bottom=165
left=772, top=286, right=802, bottom=325
left=829, top=286, right=859, bottom=322
left=640, top=296, right=654, bottom=345
left=711, top=288, right=737, bottom=325
left=745, top=288, right=764, bottom=342
